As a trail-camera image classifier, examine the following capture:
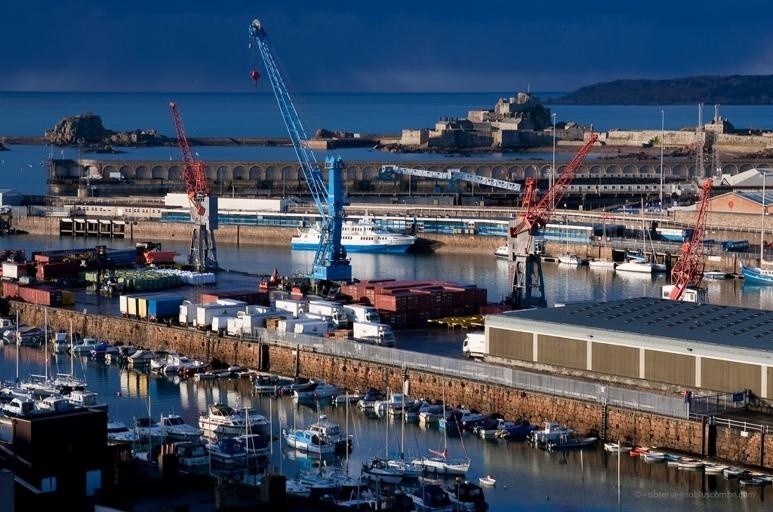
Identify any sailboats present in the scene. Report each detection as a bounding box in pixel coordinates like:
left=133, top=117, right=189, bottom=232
left=488, top=170, right=772, bottom=289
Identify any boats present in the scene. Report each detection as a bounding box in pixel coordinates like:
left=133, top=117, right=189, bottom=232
left=288, top=207, right=416, bottom=256
left=0, top=293, right=773, bottom=512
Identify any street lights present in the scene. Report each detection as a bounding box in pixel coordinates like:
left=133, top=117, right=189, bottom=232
left=550, top=111, right=561, bottom=209
left=658, top=108, right=666, bottom=205
